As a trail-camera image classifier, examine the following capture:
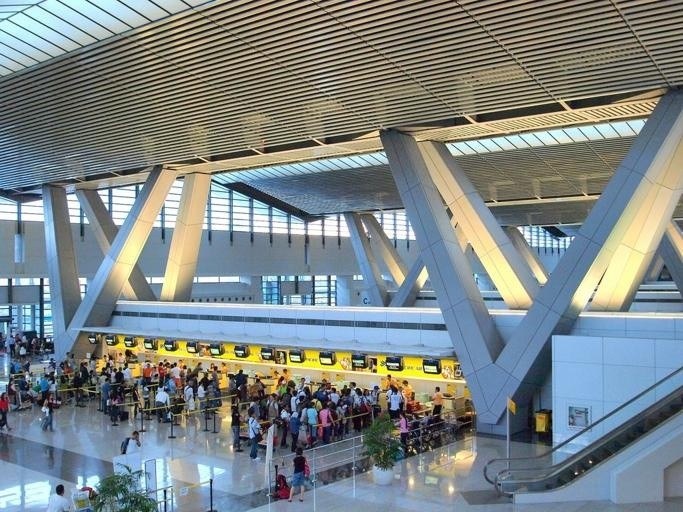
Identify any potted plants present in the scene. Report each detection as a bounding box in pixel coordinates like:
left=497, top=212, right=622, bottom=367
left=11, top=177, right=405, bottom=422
left=359, top=409, right=406, bottom=487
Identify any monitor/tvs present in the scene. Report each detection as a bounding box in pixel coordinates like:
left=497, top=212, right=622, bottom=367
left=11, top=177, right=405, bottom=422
left=352, top=355, right=368, bottom=368
left=422, top=360, right=441, bottom=374
left=319, top=352, right=335, bottom=365
left=234, top=346, right=245, bottom=357
left=386, top=357, right=403, bottom=371
left=289, top=350, right=306, bottom=363
left=88, top=335, right=173, bottom=351
left=210, top=344, right=221, bottom=355
left=261, top=349, right=272, bottom=360
left=187, top=342, right=197, bottom=353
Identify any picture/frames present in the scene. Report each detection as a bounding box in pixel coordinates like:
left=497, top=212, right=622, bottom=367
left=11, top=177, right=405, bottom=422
left=565, top=403, right=592, bottom=431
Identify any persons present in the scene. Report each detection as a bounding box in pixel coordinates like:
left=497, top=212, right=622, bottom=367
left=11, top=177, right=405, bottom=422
left=431, top=386, right=443, bottom=428
left=419, top=412, right=429, bottom=431
left=286, top=446, right=306, bottom=503
left=0, top=327, right=417, bottom=461
left=81, top=486, right=97, bottom=499
left=398, top=459, right=409, bottom=496
left=409, top=412, right=419, bottom=423
left=408, top=447, right=442, bottom=475
left=45, top=484, right=71, bottom=512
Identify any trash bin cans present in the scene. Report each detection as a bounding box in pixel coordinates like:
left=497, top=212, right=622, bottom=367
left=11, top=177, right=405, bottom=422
left=373, top=406, right=380, bottom=417
left=535, top=408, right=551, bottom=441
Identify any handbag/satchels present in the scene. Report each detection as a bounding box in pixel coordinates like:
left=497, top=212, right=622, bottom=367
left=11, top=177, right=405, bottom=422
left=275, top=418, right=285, bottom=427
left=327, top=413, right=337, bottom=422
left=120, top=438, right=129, bottom=454
left=170, top=398, right=185, bottom=414
left=318, top=428, right=323, bottom=438
left=305, top=463, right=310, bottom=474
left=360, top=404, right=367, bottom=412
left=255, top=433, right=263, bottom=442
left=278, top=475, right=290, bottom=499
